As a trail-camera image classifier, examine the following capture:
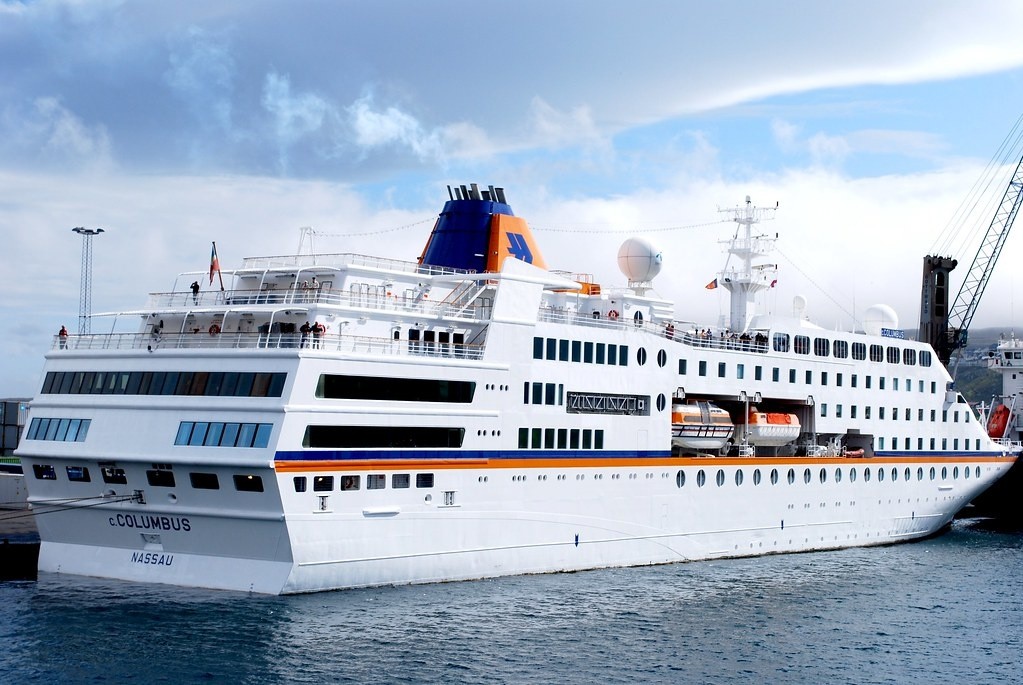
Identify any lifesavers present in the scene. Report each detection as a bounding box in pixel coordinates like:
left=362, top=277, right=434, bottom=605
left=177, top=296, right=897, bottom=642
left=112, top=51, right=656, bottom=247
left=609, top=309, right=618, bottom=319
left=209, top=325, right=220, bottom=337
left=317, top=324, right=326, bottom=335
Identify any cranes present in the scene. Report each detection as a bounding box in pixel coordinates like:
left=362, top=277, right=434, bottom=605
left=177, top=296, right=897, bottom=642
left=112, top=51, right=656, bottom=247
left=928, top=155, right=1023, bottom=368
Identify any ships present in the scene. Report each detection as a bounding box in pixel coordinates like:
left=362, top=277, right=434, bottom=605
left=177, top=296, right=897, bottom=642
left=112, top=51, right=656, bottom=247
left=13, top=180, right=1023, bottom=597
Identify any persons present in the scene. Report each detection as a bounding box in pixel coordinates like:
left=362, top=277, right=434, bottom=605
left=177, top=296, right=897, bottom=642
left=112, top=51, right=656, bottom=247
left=302, top=277, right=320, bottom=302
left=59, top=325, right=67, bottom=349
left=154, top=320, right=164, bottom=334
left=664, top=323, right=790, bottom=354
left=300, top=321, right=323, bottom=349
left=190, top=281, right=199, bottom=306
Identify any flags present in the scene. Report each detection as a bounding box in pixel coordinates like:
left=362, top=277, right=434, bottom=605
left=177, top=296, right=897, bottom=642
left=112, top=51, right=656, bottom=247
left=209, top=245, right=220, bottom=286
left=704, top=278, right=718, bottom=290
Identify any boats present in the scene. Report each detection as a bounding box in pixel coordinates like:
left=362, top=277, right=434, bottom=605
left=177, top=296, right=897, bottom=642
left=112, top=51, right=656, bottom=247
left=0, top=461, right=84, bottom=511
left=987, top=404, right=1015, bottom=438
left=672, top=398, right=737, bottom=451
left=966, top=329, right=1023, bottom=519
left=739, top=406, right=802, bottom=447
